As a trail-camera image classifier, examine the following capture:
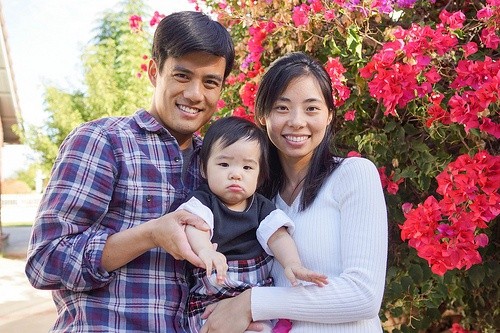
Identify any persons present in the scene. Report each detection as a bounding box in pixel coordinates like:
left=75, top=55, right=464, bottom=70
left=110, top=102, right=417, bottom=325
left=184, top=116, right=328, bottom=333
left=26, top=11, right=234, bottom=333
left=200, top=53, right=388, bottom=333
